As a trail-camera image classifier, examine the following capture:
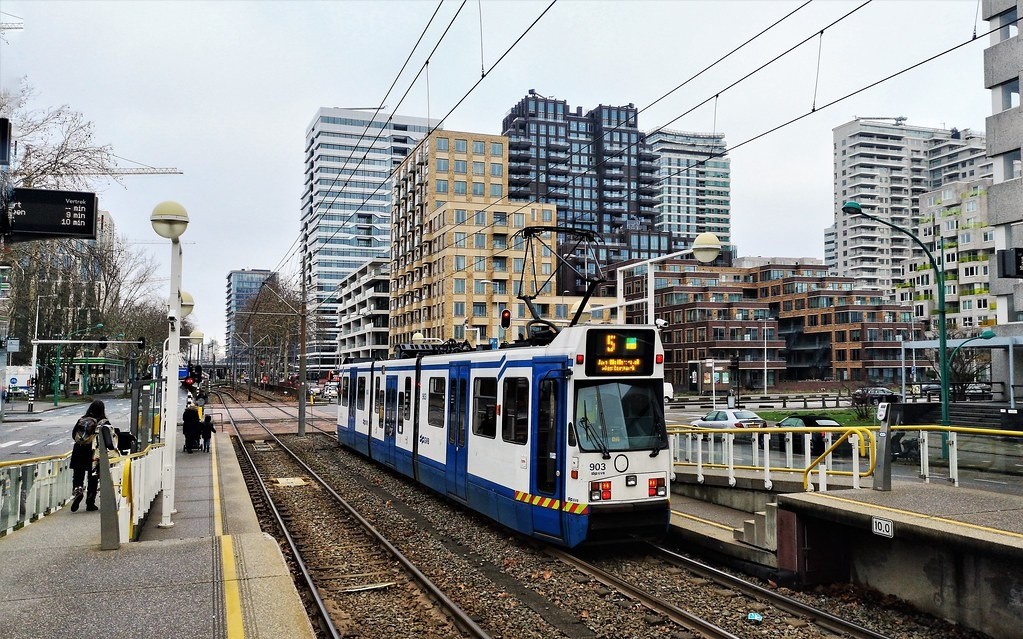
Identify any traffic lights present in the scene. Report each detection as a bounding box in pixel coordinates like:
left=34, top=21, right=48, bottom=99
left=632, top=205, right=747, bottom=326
left=500, top=310, right=511, bottom=331
left=216, top=368, right=223, bottom=379
left=259, top=354, right=265, bottom=367
left=727, top=357, right=740, bottom=371
left=186, top=377, right=193, bottom=385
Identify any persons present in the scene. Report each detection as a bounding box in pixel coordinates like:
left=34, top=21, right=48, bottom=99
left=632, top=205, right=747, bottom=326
left=182, top=404, right=201, bottom=454
left=69, top=400, right=112, bottom=512
left=200, top=416, right=216, bottom=452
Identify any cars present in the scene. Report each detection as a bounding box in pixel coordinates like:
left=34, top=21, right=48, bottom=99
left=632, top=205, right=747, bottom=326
left=309, top=382, right=340, bottom=399
left=689, top=409, right=768, bottom=442
left=852, top=387, right=904, bottom=405
left=966, top=384, right=991, bottom=391
left=240, top=370, right=251, bottom=382
left=758, top=415, right=856, bottom=458
left=259, top=371, right=320, bottom=390
left=920, top=384, right=955, bottom=395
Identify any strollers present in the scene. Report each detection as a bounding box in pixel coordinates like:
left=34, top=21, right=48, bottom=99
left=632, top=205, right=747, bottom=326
left=91, top=427, right=138, bottom=496
left=182, top=419, right=204, bottom=453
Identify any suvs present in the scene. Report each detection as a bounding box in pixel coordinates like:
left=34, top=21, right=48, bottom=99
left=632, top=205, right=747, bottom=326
left=663, top=381, right=674, bottom=403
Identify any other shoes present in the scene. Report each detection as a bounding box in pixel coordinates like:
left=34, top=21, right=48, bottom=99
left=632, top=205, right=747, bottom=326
left=86, top=504, right=98, bottom=512
left=72, top=492, right=83, bottom=512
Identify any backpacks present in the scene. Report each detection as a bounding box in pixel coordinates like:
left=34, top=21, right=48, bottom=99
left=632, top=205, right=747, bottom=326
left=74, top=416, right=108, bottom=444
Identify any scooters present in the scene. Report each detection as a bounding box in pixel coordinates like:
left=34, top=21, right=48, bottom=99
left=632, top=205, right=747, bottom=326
left=889, top=429, right=921, bottom=468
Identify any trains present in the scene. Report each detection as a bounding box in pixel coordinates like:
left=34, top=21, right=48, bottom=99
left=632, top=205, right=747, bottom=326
left=336, top=219, right=675, bottom=550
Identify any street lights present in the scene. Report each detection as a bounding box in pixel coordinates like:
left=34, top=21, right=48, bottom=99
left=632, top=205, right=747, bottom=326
left=757, top=317, right=774, bottom=398
left=67, top=334, right=126, bottom=397
left=480, top=280, right=500, bottom=350
left=149, top=200, right=204, bottom=526
left=37, top=324, right=105, bottom=406
left=616, top=233, right=722, bottom=320
left=842, top=201, right=998, bottom=460
left=27, top=294, right=59, bottom=413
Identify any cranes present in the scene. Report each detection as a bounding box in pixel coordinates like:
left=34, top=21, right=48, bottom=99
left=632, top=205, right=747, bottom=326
left=1, top=144, right=184, bottom=185
